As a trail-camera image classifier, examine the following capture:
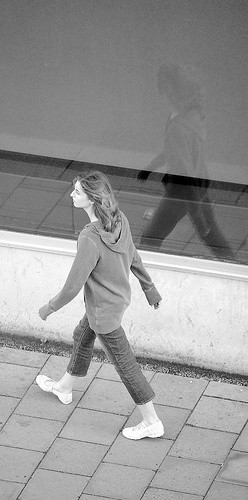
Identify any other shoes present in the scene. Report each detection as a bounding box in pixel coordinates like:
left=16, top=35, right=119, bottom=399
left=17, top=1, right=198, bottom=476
left=36, top=374, right=72, bottom=405
left=122, top=421, right=165, bottom=439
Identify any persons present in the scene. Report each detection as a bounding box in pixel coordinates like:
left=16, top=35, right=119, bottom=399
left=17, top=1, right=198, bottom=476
left=36, top=170, right=165, bottom=440
left=138, top=61, right=235, bottom=260
